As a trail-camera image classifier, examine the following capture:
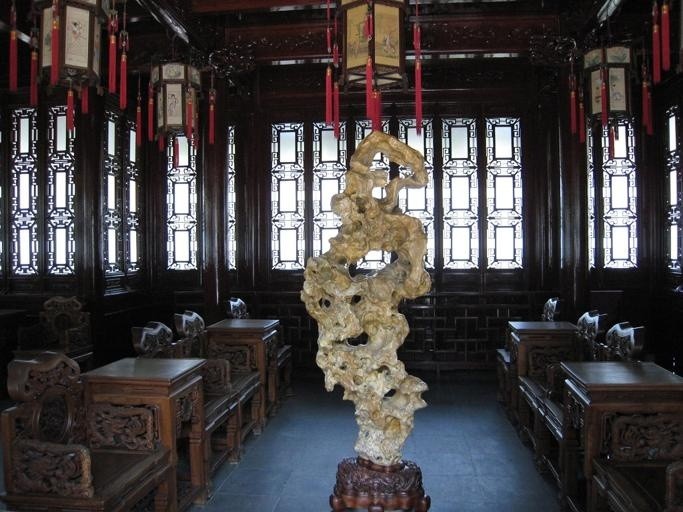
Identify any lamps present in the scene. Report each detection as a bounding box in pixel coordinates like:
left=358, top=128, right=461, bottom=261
left=582, top=15, right=634, bottom=131
left=151, top=46, right=201, bottom=137
left=38, top=0, right=109, bottom=87
left=338, top=0, right=409, bottom=92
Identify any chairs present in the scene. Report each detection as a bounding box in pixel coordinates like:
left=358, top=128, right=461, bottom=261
left=174, top=310, right=264, bottom=457
left=224, top=296, right=292, bottom=401
left=496, top=297, right=682, bottom=511
left=131, top=320, right=239, bottom=497
left=1, top=350, right=173, bottom=510
left=17, top=296, right=94, bottom=371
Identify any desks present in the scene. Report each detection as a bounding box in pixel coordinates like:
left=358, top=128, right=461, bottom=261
left=208, top=318, right=281, bottom=423
left=2, top=308, right=25, bottom=326
left=80, top=357, right=209, bottom=510
